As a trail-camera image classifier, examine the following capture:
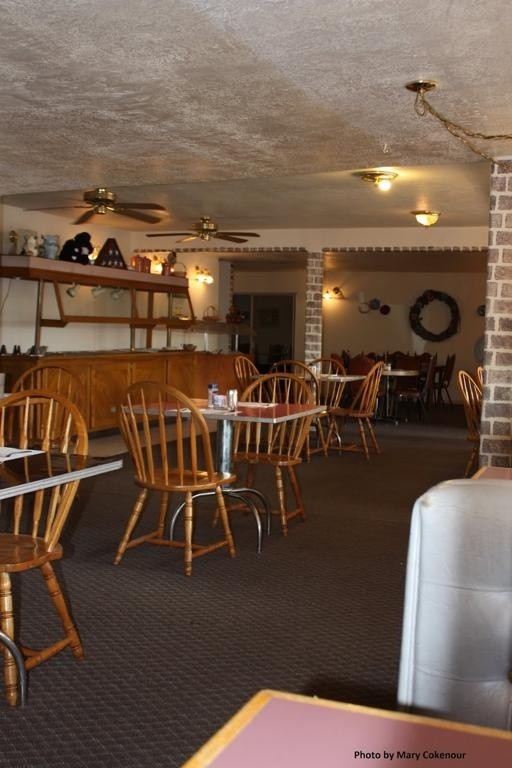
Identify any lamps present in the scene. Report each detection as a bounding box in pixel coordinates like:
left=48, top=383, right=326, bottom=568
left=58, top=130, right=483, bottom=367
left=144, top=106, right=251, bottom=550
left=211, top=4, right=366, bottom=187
left=196, top=266, right=213, bottom=284
left=353, top=171, right=398, bottom=191
left=323, top=287, right=349, bottom=300
left=411, top=211, right=441, bottom=226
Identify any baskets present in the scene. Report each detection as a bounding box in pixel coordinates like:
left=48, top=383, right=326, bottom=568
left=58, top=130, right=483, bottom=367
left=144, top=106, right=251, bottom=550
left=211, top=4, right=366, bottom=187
left=169, top=261, right=187, bottom=279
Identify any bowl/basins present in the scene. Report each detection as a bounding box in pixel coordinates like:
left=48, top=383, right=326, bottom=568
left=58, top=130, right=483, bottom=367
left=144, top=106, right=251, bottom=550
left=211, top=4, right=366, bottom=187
left=181, top=343, right=197, bottom=351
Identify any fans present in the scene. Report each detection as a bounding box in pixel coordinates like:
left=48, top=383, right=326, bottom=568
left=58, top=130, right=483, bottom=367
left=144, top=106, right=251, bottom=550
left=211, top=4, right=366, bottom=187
left=22, top=188, right=166, bottom=225
left=145, top=216, right=261, bottom=243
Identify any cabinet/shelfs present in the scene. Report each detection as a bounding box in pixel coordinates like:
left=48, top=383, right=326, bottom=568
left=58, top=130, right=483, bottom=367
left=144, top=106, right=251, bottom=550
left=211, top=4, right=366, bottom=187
left=0, top=255, right=198, bottom=351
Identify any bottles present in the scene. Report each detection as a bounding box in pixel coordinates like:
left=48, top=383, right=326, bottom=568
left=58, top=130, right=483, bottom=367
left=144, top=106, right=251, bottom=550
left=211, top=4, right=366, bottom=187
left=207, top=383, right=238, bottom=410
